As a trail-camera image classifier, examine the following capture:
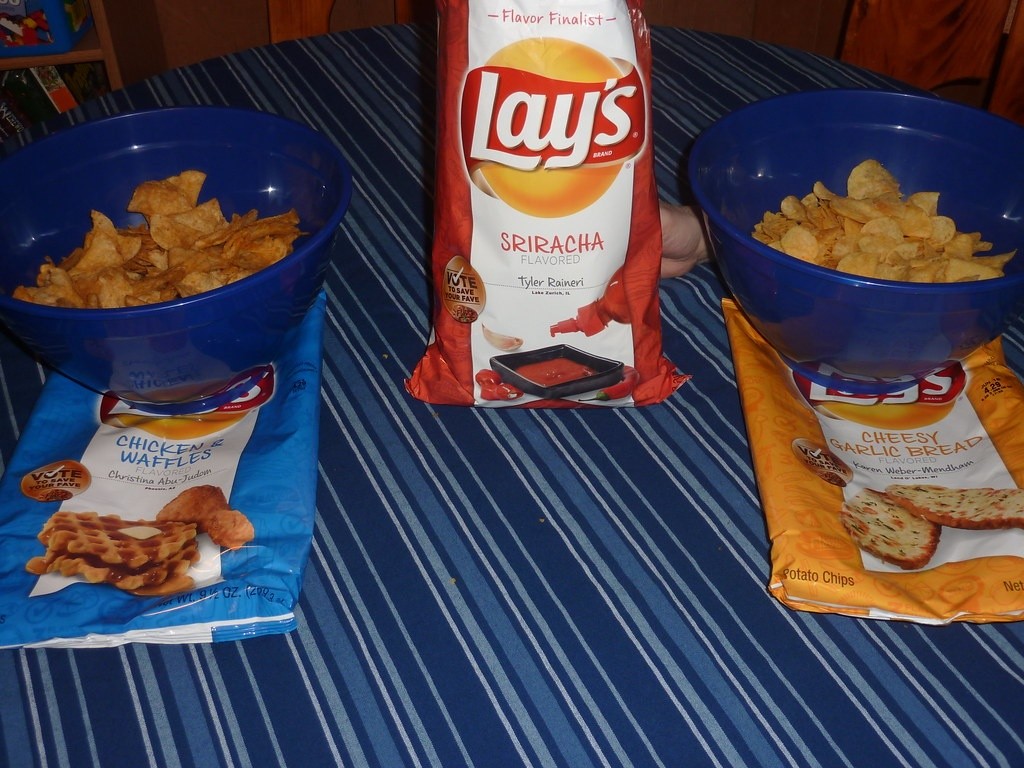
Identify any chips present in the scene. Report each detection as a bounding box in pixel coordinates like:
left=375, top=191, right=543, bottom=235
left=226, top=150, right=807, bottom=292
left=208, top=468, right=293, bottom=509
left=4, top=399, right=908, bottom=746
left=749, top=156, right=1017, bottom=284
left=12, top=170, right=300, bottom=310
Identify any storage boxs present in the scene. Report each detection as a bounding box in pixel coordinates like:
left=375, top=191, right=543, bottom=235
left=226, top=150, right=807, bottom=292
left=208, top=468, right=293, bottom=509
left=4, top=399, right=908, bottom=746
left=0, top=0, right=93, bottom=60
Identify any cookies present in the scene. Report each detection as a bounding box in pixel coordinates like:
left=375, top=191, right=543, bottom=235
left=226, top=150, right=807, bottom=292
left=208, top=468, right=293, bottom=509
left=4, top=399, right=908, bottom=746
left=24, top=509, right=199, bottom=590
left=840, top=488, right=945, bottom=570
left=887, top=482, right=1024, bottom=530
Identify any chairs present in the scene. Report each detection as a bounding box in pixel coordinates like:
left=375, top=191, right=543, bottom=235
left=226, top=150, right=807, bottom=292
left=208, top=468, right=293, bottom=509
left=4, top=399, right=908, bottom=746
left=267, top=0, right=436, bottom=46
left=838, top=0, right=1024, bottom=123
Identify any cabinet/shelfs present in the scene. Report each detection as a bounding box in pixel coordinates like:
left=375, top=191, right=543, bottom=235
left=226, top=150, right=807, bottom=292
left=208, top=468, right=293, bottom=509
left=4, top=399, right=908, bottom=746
left=0, top=0, right=165, bottom=93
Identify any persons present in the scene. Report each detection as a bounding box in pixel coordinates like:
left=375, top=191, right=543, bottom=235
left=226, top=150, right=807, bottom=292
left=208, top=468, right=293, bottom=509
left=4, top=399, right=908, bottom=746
left=658, top=200, right=709, bottom=278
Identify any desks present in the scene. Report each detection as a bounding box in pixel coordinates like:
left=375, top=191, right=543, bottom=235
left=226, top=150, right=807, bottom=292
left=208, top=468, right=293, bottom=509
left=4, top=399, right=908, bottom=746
left=0, top=24, right=1024, bottom=768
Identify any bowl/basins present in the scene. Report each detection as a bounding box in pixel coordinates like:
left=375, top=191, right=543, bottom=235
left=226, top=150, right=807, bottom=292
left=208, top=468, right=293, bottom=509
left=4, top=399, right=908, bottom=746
left=689, top=90, right=1024, bottom=394
left=1, top=106, right=350, bottom=418
